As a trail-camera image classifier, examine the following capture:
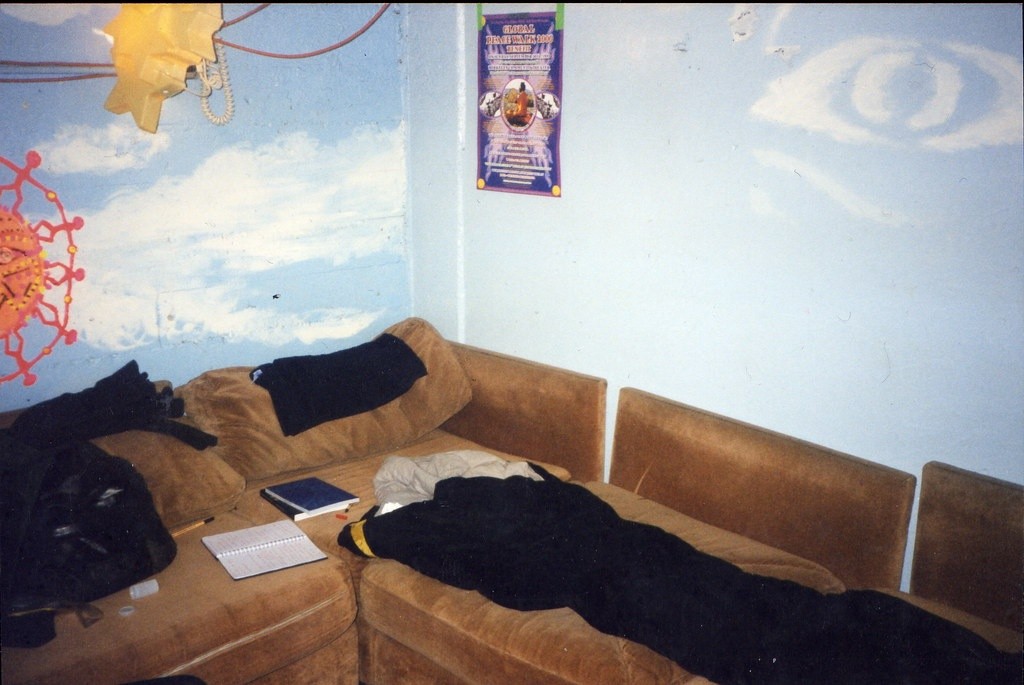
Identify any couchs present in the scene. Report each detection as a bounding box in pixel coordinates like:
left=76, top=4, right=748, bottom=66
left=1, top=337, right=1022, bottom=685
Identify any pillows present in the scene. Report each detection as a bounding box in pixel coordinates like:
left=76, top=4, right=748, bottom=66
left=85, top=433, right=247, bottom=532
left=188, top=314, right=473, bottom=480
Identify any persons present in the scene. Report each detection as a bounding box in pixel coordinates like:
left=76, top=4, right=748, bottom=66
left=512, top=82, right=528, bottom=117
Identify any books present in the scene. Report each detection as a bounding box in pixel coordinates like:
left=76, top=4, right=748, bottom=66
left=260, top=476, right=361, bottom=523
left=201, top=519, right=328, bottom=581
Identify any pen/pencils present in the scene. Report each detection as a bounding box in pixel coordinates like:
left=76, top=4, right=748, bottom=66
left=171, top=516, right=215, bottom=537
left=345, top=503, right=353, bottom=513
